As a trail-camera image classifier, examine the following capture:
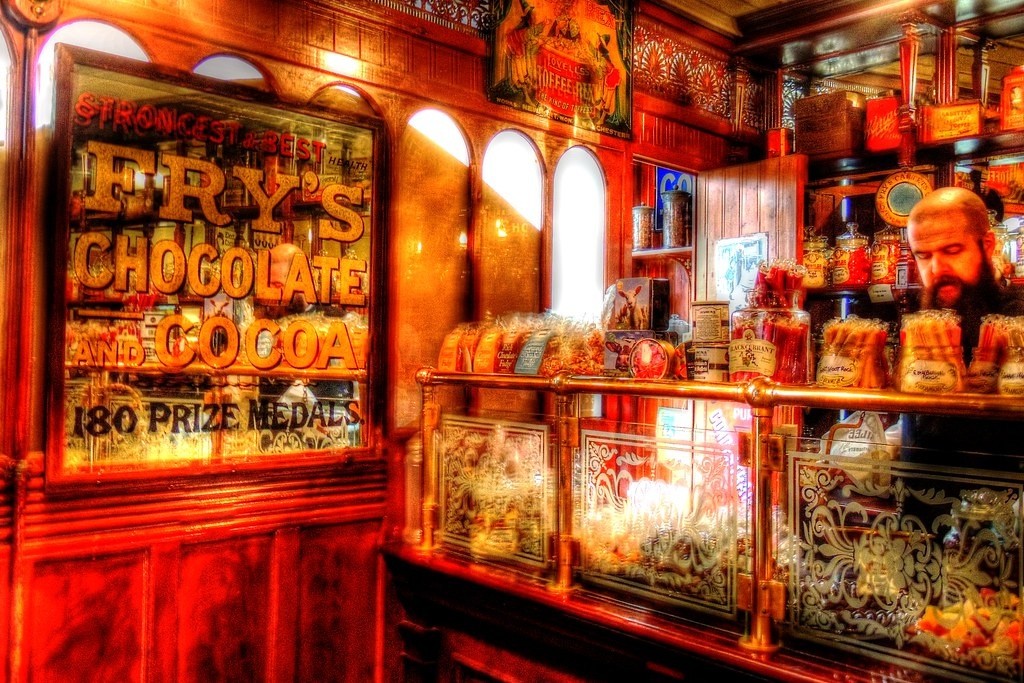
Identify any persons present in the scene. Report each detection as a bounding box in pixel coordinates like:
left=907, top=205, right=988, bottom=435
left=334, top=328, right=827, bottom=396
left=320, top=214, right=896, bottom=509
left=817, top=187, right=1024, bottom=575
